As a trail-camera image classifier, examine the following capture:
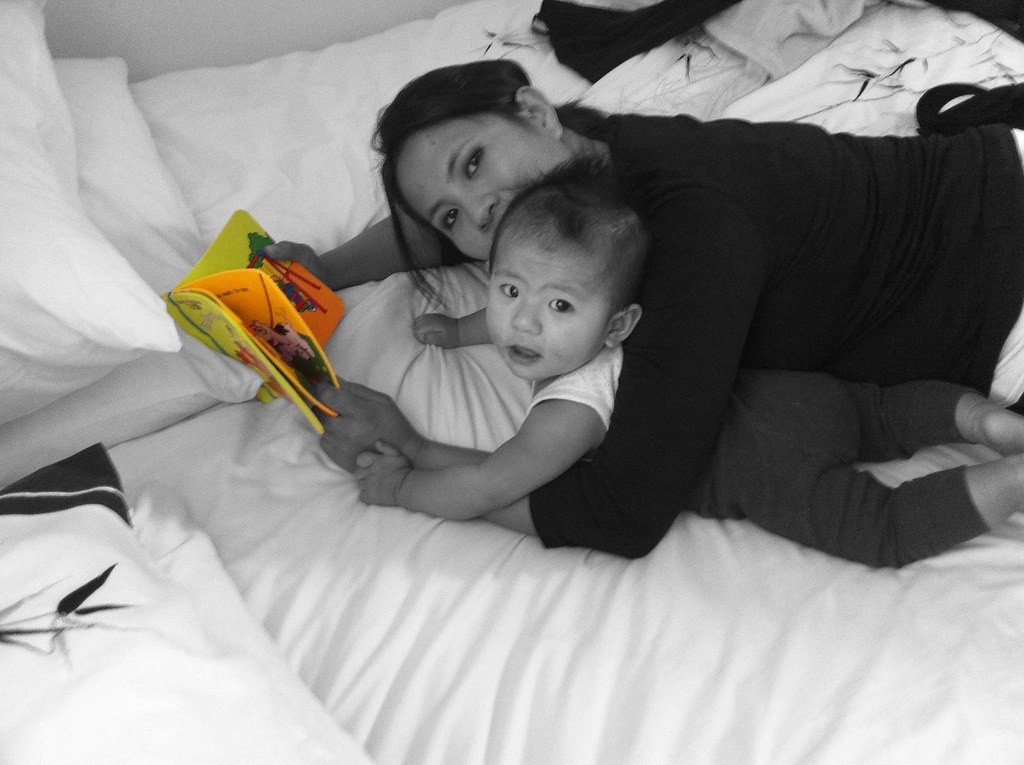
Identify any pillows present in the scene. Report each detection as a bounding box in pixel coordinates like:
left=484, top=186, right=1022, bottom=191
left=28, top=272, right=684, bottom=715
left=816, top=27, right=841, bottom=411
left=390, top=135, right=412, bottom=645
left=0, top=443, right=376, bottom=765
left=0, top=56, right=267, bottom=492
left=0, top=0, right=183, bottom=432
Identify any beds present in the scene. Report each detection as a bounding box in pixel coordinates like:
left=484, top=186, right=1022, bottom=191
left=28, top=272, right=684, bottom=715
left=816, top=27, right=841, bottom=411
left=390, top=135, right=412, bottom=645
left=0, top=0, right=1024, bottom=765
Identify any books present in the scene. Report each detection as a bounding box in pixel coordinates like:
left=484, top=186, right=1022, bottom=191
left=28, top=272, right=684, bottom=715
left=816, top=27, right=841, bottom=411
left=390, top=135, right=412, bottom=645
left=161, top=210, right=345, bottom=435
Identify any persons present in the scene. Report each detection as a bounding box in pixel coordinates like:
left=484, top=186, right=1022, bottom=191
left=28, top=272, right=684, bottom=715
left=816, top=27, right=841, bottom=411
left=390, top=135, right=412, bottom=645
left=265, top=60, right=1024, bottom=567
